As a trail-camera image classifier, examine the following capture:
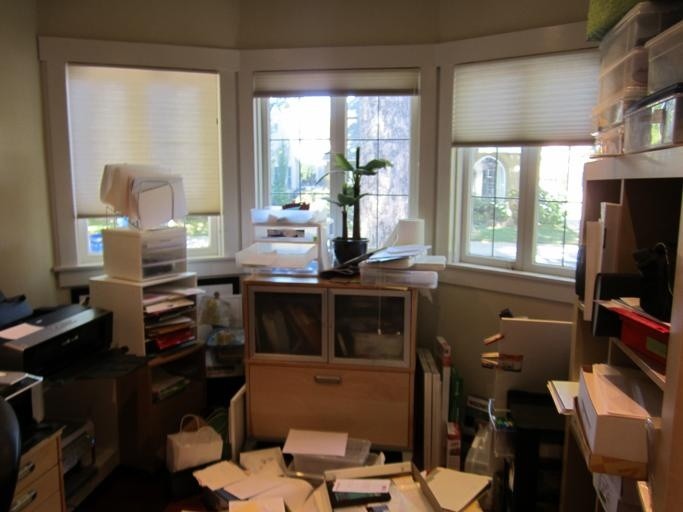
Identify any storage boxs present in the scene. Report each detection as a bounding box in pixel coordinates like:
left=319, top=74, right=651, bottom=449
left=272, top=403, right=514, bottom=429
left=102, top=224, right=187, bottom=282
left=235, top=215, right=329, bottom=278
left=286, top=438, right=380, bottom=488
left=590, top=0, right=683, bottom=160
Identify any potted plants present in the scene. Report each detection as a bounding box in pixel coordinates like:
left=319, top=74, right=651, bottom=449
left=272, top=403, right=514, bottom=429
left=314, top=147, right=394, bottom=269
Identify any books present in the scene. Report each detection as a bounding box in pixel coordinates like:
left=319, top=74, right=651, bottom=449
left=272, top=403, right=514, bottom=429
left=336, top=326, right=350, bottom=357
left=143, top=283, right=207, bottom=405
left=256, top=303, right=321, bottom=357
left=321, top=242, right=432, bottom=280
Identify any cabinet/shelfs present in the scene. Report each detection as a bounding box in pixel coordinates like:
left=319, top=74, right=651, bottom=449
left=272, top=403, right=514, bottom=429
left=242, top=272, right=419, bottom=462
left=559, top=145, right=683, bottom=512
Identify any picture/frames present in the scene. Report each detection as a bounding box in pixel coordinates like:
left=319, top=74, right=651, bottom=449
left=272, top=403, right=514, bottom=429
left=197, top=276, right=240, bottom=298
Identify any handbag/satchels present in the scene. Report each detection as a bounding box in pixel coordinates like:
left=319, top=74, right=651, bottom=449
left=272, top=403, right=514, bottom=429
left=166, top=414, right=223, bottom=473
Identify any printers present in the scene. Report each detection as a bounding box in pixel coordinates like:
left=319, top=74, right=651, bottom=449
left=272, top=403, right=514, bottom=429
left=0, top=295, right=113, bottom=379
left=0, top=370, right=47, bottom=438
